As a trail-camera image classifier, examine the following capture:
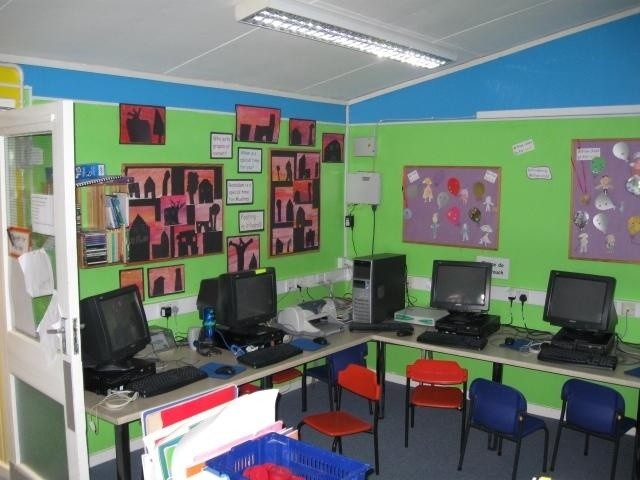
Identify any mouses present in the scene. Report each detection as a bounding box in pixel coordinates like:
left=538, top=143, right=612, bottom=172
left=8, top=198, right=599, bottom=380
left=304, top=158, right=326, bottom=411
left=397, top=329, right=413, bottom=337
left=505, top=337, right=515, bottom=345
left=215, top=365, right=236, bottom=375
left=313, top=337, right=328, bottom=345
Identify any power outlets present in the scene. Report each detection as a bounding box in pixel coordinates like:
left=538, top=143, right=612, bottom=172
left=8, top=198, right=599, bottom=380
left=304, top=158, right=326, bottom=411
left=295, top=278, right=307, bottom=290
left=621, top=302, right=636, bottom=316
left=160, top=303, right=174, bottom=317
left=518, top=288, right=529, bottom=303
left=508, top=288, right=517, bottom=301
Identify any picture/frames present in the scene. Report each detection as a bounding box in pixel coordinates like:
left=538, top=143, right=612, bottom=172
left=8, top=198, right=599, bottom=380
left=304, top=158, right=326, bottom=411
left=287, top=117, right=316, bottom=147
left=147, top=264, right=187, bottom=297
left=120, top=105, right=167, bottom=145
left=321, top=132, right=344, bottom=163
left=402, top=165, right=501, bottom=250
left=270, top=148, right=321, bottom=255
left=227, top=234, right=260, bottom=273
left=122, top=162, right=224, bottom=267
left=236, top=105, right=281, bottom=145
left=119, top=268, right=146, bottom=301
left=568, top=137, right=640, bottom=264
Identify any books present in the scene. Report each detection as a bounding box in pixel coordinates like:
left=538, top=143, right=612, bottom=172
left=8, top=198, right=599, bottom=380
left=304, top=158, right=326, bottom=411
left=140, top=382, right=299, bottom=480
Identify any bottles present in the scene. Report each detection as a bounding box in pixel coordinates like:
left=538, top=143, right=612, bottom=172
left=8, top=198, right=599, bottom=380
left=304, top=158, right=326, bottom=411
left=203, top=306, right=217, bottom=343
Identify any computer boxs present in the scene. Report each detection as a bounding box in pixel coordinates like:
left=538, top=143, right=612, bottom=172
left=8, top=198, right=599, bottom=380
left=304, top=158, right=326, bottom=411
left=435, top=314, right=501, bottom=338
left=82, top=358, right=157, bottom=396
left=352, top=253, right=406, bottom=324
left=551, top=327, right=615, bottom=356
left=198, top=324, right=287, bottom=352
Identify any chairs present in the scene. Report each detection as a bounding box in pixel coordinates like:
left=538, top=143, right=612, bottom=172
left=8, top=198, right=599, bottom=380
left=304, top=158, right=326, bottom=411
left=307, top=343, right=372, bottom=415
left=405, top=359, right=467, bottom=447
left=551, top=379, right=637, bottom=480
left=298, top=364, right=380, bottom=476
left=458, top=378, right=549, bottom=480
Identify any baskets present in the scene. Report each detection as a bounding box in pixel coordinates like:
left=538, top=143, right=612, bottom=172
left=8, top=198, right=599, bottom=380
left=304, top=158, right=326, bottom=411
left=204, top=433, right=373, bottom=480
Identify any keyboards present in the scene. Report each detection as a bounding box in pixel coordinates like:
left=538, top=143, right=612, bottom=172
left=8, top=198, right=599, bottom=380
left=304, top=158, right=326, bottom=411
left=537, top=345, right=618, bottom=371
left=416, top=331, right=489, bottom=350
left=349, top=323, right=414, bottom=332
left=124, top=365, right=209, bottom=398
left=236, top=343, right=304, bottom=369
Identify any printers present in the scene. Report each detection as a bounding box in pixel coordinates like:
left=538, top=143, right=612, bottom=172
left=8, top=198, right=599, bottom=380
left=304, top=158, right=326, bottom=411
left=272, top=298, right=346, bottom=338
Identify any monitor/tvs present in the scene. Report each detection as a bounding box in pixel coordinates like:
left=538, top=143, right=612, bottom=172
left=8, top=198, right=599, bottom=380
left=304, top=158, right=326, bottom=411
left=429, top=260, right=493, bottom=325
left=196, top=266, right=278, bottom=334
left=79, top=283, right=152, bottom=372
left=543, top=270, right=617, bottom=343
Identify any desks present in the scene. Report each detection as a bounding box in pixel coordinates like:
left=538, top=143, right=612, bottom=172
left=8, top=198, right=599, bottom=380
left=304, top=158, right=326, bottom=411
left=85, top=295, right=372, bottom=480
left=372, top=325, right=640, bottom=418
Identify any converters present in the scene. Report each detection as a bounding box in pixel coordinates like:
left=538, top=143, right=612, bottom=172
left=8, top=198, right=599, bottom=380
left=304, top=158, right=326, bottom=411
left=161, top=307, right=171, bottom=317
left=345, top=215, right=354, bottom=228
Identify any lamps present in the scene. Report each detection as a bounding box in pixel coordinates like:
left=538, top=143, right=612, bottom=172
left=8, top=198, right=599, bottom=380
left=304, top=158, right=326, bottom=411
left=233, top=0, right=457, bottom=71
